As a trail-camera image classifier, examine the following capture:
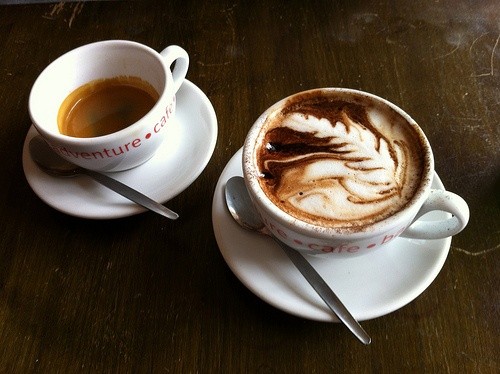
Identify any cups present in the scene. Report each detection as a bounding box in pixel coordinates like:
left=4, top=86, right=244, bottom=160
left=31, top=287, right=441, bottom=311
left=28, top=39, right=189, bottom=173
left=241, top=88, right=470, bottom=260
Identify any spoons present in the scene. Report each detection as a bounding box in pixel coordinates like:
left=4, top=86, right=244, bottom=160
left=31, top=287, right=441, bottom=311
left=224, top=176, right=372, bottom=345
left=28, top=135, right=179, bottom=220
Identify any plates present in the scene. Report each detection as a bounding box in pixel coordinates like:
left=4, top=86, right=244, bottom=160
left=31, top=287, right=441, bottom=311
left=212, top=145, right=453, bottom=323
left=22, top=78, right=218, bottom=219
